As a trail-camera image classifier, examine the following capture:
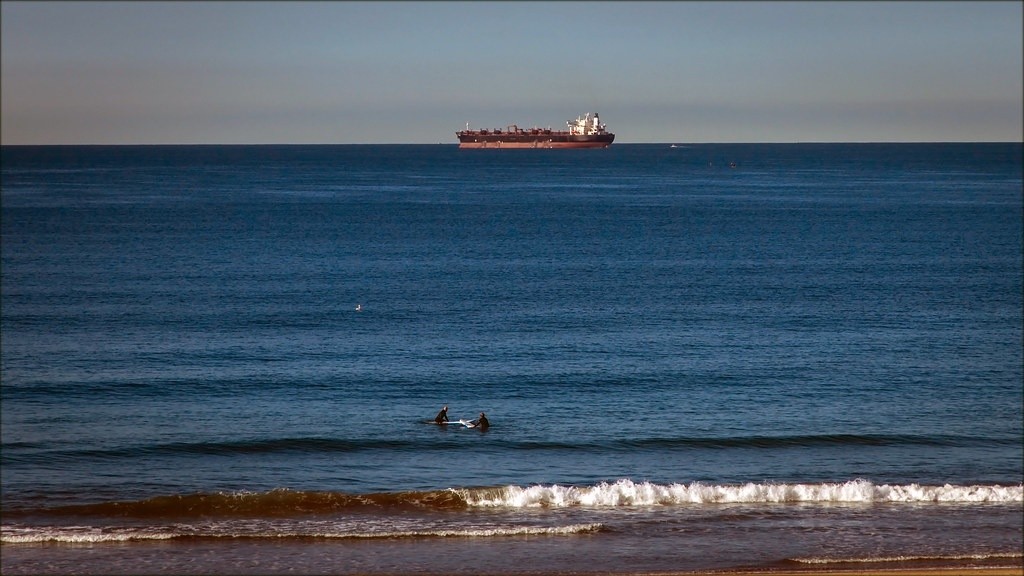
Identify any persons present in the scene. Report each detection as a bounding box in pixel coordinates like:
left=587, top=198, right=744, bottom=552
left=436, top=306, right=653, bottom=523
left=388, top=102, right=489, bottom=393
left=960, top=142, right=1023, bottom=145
left=435, top=406, right=449, bottom=425
left=469, top=412, right=490, bottom=433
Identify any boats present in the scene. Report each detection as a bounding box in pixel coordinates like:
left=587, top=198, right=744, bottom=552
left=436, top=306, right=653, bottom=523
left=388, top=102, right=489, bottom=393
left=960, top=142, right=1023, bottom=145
left=454, top=112, right=616, bottom=151
